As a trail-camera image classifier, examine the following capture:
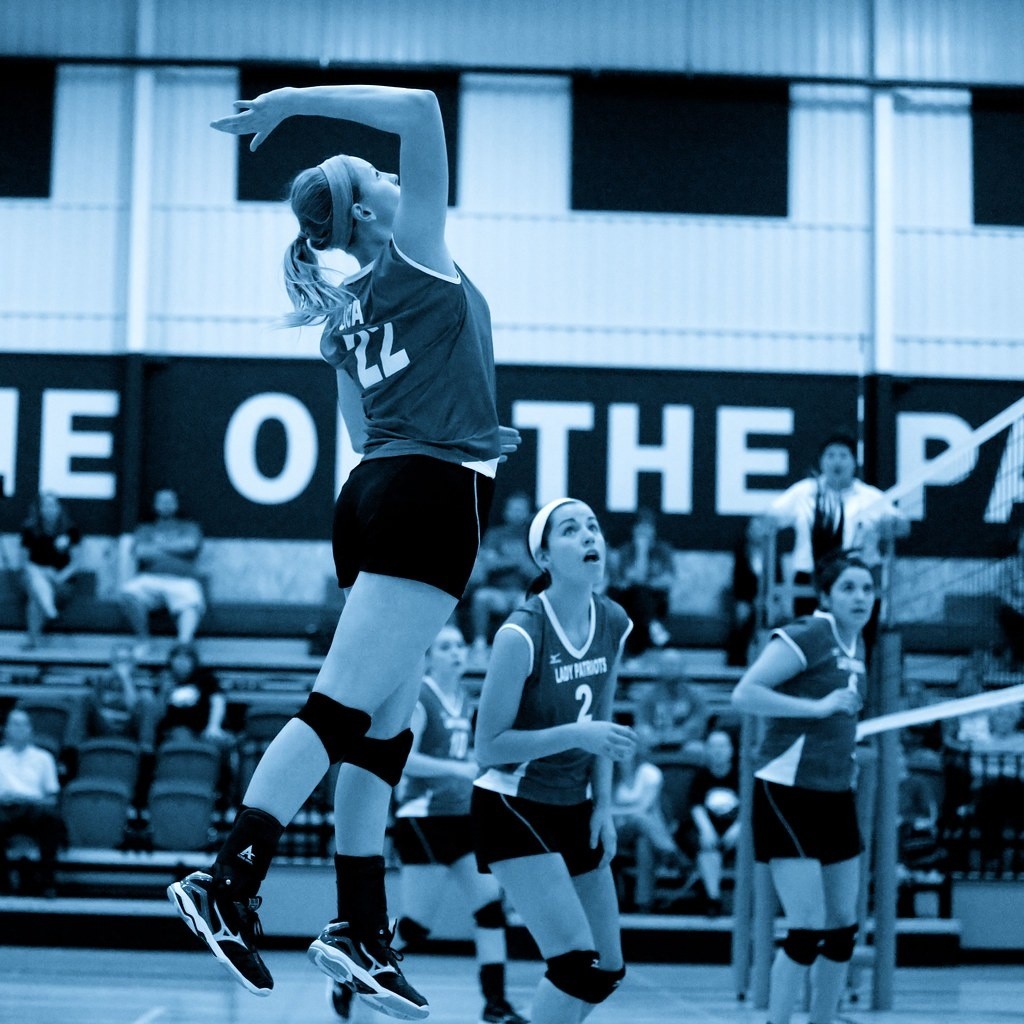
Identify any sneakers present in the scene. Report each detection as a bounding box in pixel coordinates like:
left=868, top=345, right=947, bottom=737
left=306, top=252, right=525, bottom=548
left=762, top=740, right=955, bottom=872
left=477, top=1000, right=532, bottom=1024
left=323, top=970, right=355, bottom=1022
left=304, top=916, right=434, bottom=1020
left=166, top=861, right=277, bottom=996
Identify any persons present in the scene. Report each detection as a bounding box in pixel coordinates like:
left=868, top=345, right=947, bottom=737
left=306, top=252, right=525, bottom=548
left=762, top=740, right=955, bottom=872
left=731, top=558, right=875, bottom=1024
left=898, top=670, right=1024, bottom=871
left=0, top=710, right=60, bottom=898
left=637, top=648, right=706, bottom=774
left=678, top=728, right=741, bottom=916
left=151, top=644, right=232, bottom=749
left=119, top=487, right=206, bottom=658
left=615, top=754, right=690, bottom=909
left=469, top=498, right=633, bottom=1024
left=728, top=518, right=783, bottom=667
left=165, top=83, right=523, bottom=1019
left=325, top=623, right=527, bottom=1024
left=614, top=514, right=675, bottom=670
left=463, top=493, right=541, bottom=652
left=77, top=643, right=158, bottom=748
left=18, top=493, right=81, bottom=649
left=765, top=435, right=910, bottom=664
left=991, top=535, right=1024, bottom=671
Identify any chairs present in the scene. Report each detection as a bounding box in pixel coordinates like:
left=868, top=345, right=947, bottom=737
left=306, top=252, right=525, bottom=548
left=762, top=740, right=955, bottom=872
left=0, top=575, right=1024, bottom=961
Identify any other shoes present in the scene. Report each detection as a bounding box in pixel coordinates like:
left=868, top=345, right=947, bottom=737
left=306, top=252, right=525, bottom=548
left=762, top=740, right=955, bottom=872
left=632, top=901, right=655, bottom=916
left=663, top=848, right=701, bottom=893
left=709, top=901, right=723, bottom=919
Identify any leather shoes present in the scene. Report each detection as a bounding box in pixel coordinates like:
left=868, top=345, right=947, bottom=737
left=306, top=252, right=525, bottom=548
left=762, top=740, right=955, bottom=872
left=651, top=630, right=673, bottom=647
